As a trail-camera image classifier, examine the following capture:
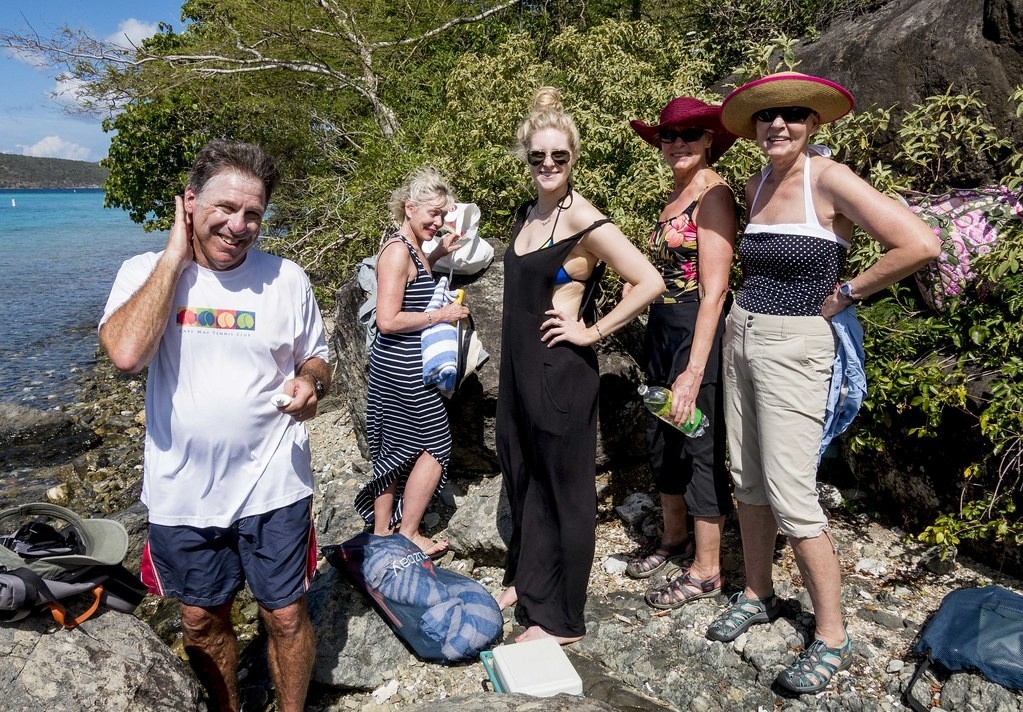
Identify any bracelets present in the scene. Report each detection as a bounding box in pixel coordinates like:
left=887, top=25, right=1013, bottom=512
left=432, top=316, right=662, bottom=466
left=596, top=323, right=604, bottom=339
left=425, top=312, right=432, bottom=327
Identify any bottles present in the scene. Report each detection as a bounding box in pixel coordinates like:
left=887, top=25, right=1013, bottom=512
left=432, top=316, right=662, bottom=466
left=637, top=385, right=709, bottom=439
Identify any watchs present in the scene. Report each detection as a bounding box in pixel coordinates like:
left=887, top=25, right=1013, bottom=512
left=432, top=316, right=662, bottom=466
left=839, top=281, right=855, bottom=302
left=303, top=370, right=324, bottom=399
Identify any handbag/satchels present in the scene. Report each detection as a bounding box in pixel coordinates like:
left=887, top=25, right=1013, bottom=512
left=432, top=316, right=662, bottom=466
left=903, top=586, right=1023, bottom=712
left=421, top=203, right=495, bottom=274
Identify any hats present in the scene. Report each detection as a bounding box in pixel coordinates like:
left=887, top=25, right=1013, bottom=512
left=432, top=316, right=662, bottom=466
left=631, top=98, right=739, bottom=164
left=0, top=502, right=129, bottom=581
left=720, top=70, right=857, bottom=140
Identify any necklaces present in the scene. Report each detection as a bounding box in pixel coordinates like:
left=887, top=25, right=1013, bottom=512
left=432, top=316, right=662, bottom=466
left=537, top=205, right=556, bottom=214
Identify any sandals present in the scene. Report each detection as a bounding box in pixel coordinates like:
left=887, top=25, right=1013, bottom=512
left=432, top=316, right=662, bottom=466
left=626, top=536, right=694, bottom=578
left=408, top=533, right=448, bottom=560
left=777, top=630, right=853, bottom=693
left=643, top=564, right=725, bottom=610
left=707, top=584, right=781, bottom=642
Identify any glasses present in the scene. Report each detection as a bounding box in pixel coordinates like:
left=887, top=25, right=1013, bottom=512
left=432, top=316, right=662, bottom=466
left=754, top=106, right=817, bottom=124
left=527, top=150, right=573, bottom=166
left=659, top=128, right=713, bottom=143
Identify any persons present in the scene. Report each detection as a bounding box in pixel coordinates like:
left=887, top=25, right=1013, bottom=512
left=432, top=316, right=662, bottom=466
left=99, top=141, right=331, bottom=712
left=351, top=166, right=469, bottom=561
left=623, top=97, right=738, bottom=606
left=707, top=72, right=941, bottom=693
left=492, top=85, right=665, bottom=642
